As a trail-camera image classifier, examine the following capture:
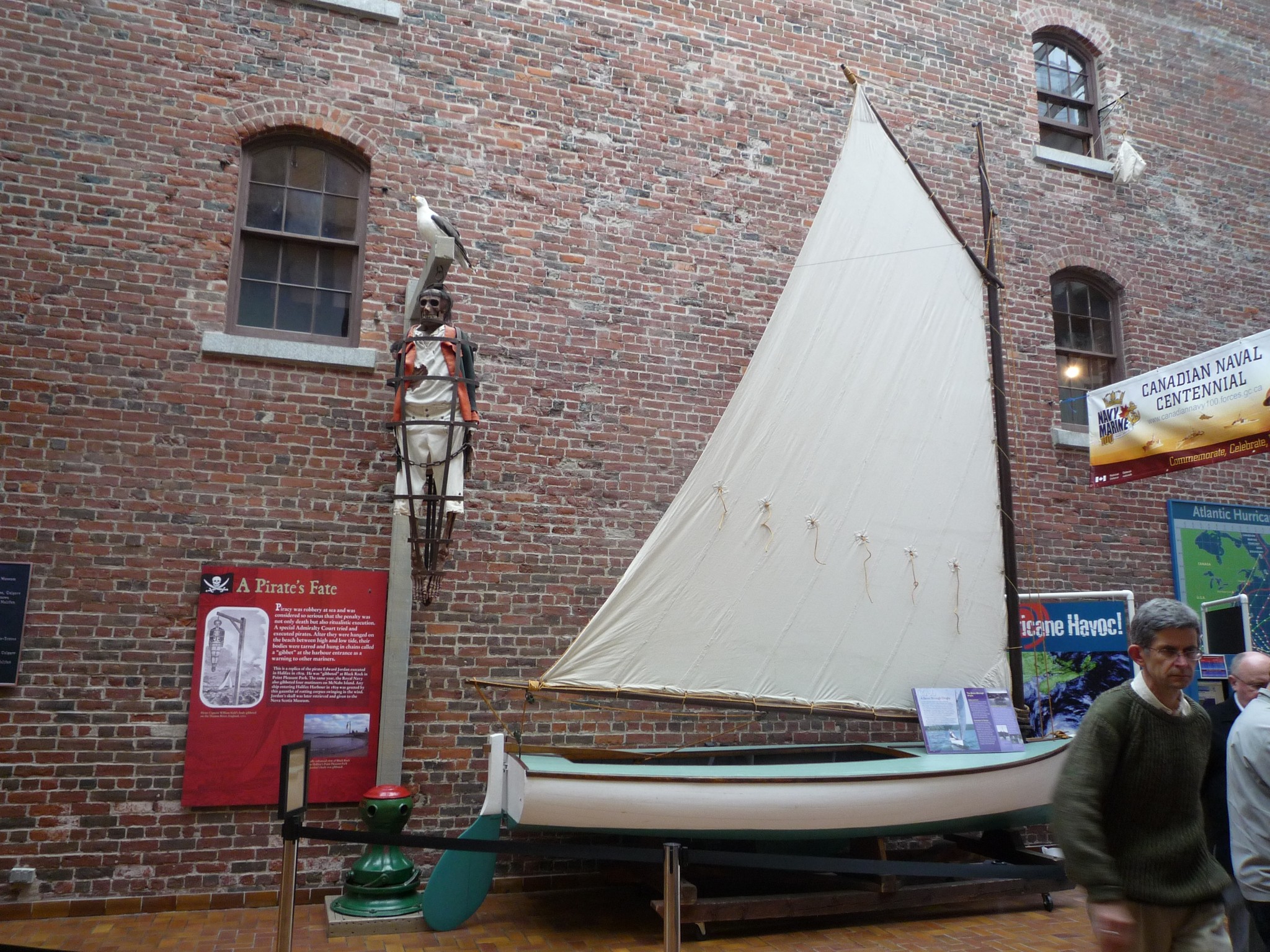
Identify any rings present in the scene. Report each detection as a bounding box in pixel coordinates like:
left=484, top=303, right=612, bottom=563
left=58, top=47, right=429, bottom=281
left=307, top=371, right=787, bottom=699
left=1101, top=929, right=1121, bottom=936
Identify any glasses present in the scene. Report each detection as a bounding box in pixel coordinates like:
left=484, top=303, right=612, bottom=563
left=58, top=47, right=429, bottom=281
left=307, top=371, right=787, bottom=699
left=1232, top=676, right=1267, bottom=690
left=1135, top=642, right=1204, bottom=660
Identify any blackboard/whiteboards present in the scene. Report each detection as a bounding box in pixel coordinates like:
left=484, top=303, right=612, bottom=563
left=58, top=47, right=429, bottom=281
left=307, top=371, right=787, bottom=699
left=0, top=560, right=34, bottom=686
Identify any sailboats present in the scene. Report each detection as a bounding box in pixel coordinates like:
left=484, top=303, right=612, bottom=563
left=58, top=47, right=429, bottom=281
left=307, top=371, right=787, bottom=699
left=422, top=64, right=1077, bottom=932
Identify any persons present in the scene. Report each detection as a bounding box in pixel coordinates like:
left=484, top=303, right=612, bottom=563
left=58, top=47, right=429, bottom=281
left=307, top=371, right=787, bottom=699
left=1049, top=598, right=1233, bottom=952
left=1201, top=651, right=1270, bottom=952
left=1227, top=678, right=1270, bottom=952
